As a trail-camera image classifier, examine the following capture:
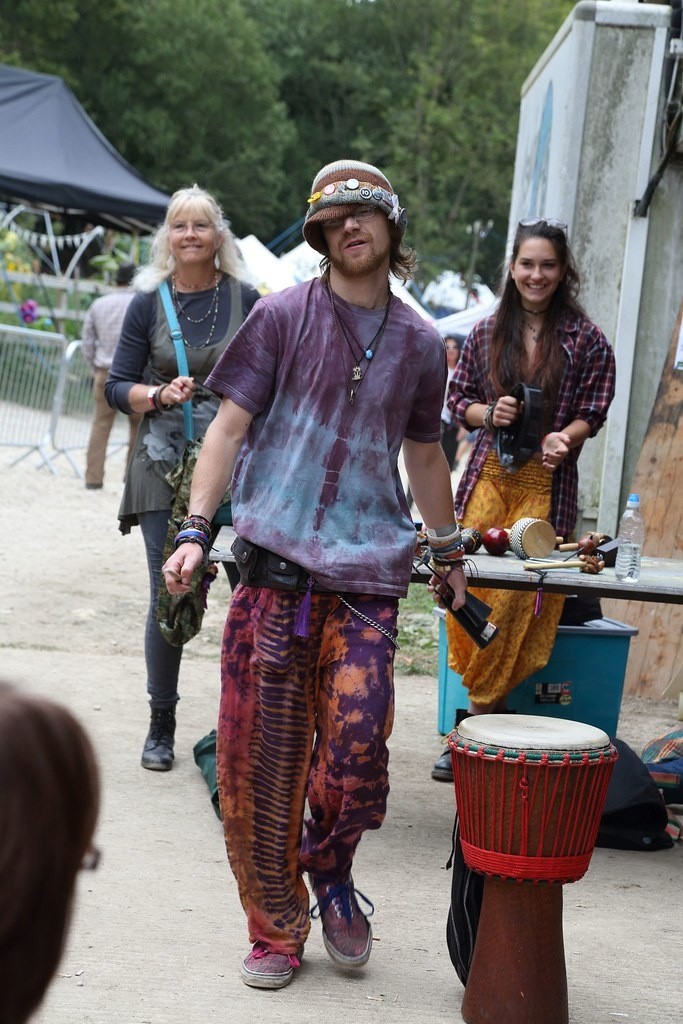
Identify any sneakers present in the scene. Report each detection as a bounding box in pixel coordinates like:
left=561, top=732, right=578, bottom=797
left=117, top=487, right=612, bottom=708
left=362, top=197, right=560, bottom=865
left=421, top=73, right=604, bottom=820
left=308, top=871, right=375, bottom=966
left=241, top=939, right=306, bottom=987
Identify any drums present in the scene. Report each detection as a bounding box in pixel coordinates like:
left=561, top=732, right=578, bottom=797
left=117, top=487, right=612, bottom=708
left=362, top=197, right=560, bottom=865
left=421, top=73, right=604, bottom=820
left=447, top=712, right=621, bottom=1024
left=509, top=517, right=557, bottom=560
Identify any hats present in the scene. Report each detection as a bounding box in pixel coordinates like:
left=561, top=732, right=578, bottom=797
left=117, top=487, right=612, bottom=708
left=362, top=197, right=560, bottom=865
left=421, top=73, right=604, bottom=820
left=302, top=160, right=407, bottom=257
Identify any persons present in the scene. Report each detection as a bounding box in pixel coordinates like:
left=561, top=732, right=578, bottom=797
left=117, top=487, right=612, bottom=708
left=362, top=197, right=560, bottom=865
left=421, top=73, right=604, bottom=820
left=408, top=336, right=478, bottom=508
left=105, top=184, right=263, bottom=772
left=163, top=161, right=468, bottom=989
left=0, top=681, right=102, bottom=1024
left=430, top=217, right=616, bottom=782
left=81, top=263, right=143, bottom=490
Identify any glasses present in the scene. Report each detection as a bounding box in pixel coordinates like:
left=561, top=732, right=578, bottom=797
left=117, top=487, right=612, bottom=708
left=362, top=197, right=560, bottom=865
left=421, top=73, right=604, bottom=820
left=170, top=219, right=215, bottom=234
left=518, top=217, right=568, bottom=242
left=70, top=842, right=100, bottom=873
left=318, top=204, right=378, bottom=228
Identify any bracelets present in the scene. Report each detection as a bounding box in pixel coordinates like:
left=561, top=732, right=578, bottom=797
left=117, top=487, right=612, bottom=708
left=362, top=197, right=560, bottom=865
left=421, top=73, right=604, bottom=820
left=427, top=526, right=479, bottom=584
left=174, top=514, right=220, bottom=557
left=146, top=383, right=168, bottom=416
left=483, top=402, right=497, bottom=432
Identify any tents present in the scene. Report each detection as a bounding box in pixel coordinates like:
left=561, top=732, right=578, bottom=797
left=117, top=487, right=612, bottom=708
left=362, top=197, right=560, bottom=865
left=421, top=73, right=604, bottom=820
left=0, top=64, right=172, bottom=266
left=238, top=217, right=434, bottom=325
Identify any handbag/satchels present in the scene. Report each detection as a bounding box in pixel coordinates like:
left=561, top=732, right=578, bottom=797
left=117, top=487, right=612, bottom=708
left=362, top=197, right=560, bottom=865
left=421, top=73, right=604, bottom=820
left=153, top=436, right=238, bottom=647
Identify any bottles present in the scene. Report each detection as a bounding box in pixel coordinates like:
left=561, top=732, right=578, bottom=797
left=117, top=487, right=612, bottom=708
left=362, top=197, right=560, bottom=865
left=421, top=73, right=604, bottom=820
left=615, top=493, right=645, bottom=583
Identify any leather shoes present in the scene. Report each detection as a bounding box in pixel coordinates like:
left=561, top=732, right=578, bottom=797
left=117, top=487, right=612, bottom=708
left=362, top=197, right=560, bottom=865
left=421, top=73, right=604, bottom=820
left=432, top=745, right=454, bottom=779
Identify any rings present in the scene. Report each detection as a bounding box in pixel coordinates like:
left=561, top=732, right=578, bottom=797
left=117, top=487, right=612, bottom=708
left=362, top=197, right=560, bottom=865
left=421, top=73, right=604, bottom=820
left=174, top=396, right=178, bottom=401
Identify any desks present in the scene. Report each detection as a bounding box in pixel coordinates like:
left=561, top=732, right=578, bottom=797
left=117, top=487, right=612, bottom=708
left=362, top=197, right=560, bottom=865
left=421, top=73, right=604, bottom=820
left=409, top=543, right=683, bottom=606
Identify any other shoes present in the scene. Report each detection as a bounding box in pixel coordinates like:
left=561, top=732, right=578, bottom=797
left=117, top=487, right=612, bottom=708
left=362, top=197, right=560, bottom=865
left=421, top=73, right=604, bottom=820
left=86, top=482, right=103, bottom=489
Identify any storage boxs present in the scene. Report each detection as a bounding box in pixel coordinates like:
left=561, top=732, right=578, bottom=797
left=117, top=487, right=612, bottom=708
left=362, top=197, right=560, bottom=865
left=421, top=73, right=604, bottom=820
left=433, top=606, right=639, bottom=739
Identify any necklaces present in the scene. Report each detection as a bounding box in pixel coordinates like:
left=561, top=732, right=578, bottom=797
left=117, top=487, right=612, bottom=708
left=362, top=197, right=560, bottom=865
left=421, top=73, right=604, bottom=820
left=523, top=318, right=539, bottom=343
left=522, top=307, right=548, bottom=315
left=325, top=277, right=391, bottom=406
left=172, top=273, right=219, bottom=350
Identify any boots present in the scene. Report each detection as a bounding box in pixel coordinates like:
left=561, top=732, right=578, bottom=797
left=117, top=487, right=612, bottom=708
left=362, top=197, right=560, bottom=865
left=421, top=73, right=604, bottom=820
left=141, top=705, right=176, bottom=771
